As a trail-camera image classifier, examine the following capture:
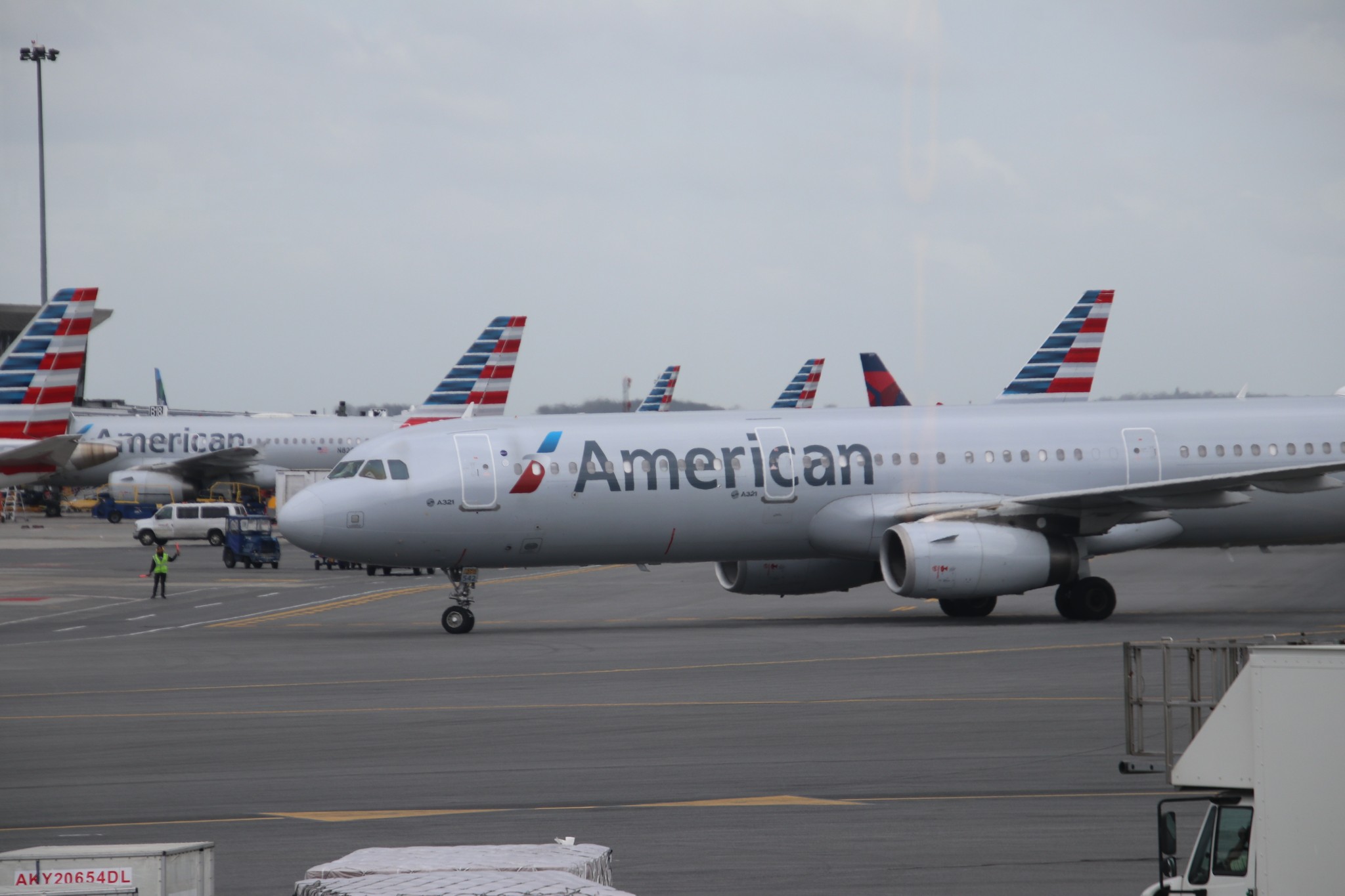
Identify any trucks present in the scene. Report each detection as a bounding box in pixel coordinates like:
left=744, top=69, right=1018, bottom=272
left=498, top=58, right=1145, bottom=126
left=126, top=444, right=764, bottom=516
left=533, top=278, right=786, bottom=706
left=91, top=496, right=159, bottom=523
left=1141, top=646, right=1345, bottom=896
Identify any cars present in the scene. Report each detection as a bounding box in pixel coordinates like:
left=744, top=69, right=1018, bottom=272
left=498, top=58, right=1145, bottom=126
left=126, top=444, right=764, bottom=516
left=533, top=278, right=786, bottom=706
left=222, top=515, right=280, bottom=569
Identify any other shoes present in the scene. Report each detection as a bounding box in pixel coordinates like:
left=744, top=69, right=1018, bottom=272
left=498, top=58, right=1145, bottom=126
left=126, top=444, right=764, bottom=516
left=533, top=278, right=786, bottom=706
left=150, top=593, right=156, bottom=599
left=160, top=593, right=166, bottom=599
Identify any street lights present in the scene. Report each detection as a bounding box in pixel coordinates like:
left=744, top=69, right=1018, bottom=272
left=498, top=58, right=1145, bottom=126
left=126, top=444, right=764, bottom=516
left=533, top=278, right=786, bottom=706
left=19, top=45, right=59, bottom=305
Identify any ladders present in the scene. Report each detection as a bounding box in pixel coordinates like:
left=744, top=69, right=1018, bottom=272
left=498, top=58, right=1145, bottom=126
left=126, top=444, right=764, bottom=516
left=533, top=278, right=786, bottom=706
left=3, top=486, right=29, bottom=522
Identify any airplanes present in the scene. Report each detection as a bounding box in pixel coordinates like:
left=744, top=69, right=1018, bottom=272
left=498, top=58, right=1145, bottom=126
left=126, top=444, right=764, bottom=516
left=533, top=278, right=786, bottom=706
left=0, top=287, right=528, bottom=518
left=274, top=290, right=1345, bottom=616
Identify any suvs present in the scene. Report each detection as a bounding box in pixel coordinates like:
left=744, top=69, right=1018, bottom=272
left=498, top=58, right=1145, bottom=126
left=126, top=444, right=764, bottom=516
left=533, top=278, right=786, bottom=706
left=135, top=502, right=246, bottom=546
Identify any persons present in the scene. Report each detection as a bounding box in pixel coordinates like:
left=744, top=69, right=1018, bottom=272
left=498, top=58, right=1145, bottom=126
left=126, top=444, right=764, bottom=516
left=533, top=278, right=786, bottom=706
left=147, top=545, right=180, bottom=599
